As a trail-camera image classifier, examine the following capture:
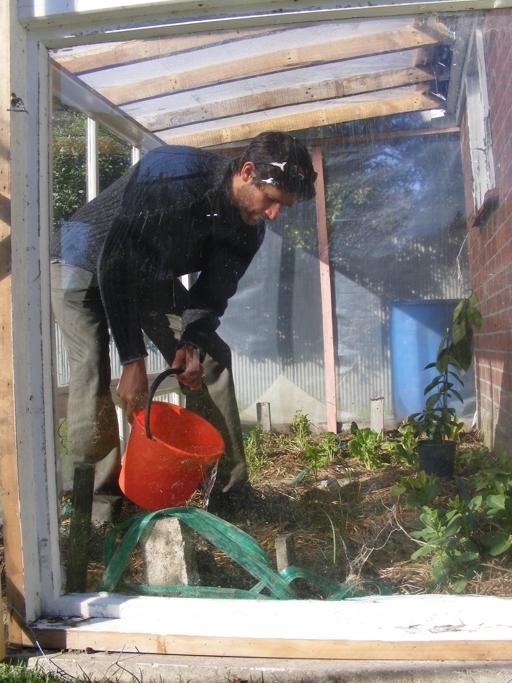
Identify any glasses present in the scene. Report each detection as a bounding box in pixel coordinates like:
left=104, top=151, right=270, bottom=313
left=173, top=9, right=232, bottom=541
left=253, top=160, right=318, bottom=186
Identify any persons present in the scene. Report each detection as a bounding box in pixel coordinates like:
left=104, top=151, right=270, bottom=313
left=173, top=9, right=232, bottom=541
left=47, top=128, right=321, bottom=530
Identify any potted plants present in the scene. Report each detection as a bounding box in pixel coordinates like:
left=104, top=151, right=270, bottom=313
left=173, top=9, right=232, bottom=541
left=407, top=289, right=477, bottom=478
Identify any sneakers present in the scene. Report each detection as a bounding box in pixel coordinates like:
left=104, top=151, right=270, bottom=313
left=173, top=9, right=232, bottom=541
left=88, top=521, right=114, bottom=562
left=208, top=486, right=283, bottom=524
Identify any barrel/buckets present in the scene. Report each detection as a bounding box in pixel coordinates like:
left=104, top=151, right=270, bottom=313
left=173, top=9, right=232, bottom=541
left=417, top=439, right=456, bottom=479
left=118, top=369, right=223, bottom=511
